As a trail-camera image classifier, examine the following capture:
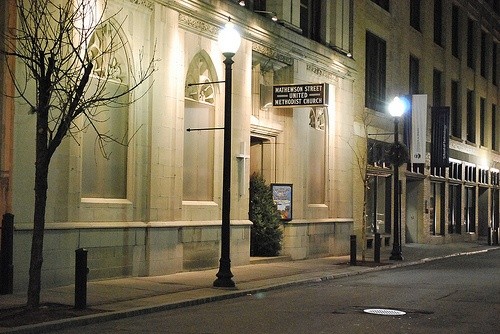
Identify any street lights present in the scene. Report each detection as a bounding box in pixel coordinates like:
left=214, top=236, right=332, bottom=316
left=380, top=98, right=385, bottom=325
left=390, top=95, right=405, bottom=260
left=214, top=22, right=242, bottom=287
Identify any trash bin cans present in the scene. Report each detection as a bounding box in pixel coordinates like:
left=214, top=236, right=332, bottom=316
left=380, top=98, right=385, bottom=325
left=488, top=226, right=500, bottom=245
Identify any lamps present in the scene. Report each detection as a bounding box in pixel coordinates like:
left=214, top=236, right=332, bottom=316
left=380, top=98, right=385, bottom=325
left=255, top=10, right=278, bottom=22
left=331, top=45, right=352, bottom=58
left=238, top=0, right=246, bottom=7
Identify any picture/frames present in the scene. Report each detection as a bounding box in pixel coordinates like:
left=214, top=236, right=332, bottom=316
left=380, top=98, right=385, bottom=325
left=271, top=183, right=293, bottom=221
